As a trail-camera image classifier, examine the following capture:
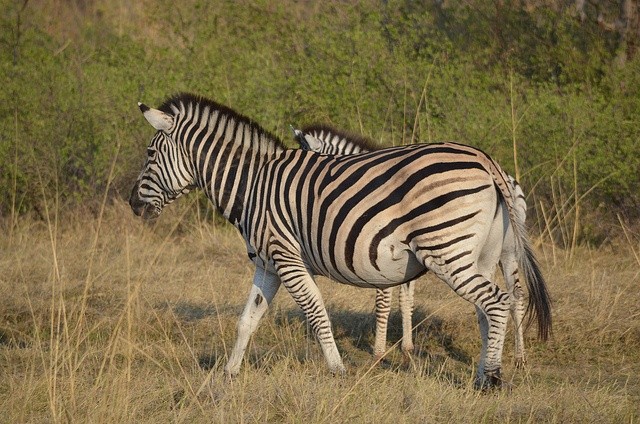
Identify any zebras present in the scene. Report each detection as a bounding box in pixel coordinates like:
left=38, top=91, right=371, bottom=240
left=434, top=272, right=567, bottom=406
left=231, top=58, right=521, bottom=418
left=291, top=123, right=527, bottom=369
left=130, top=90, right=554, bottom=396
